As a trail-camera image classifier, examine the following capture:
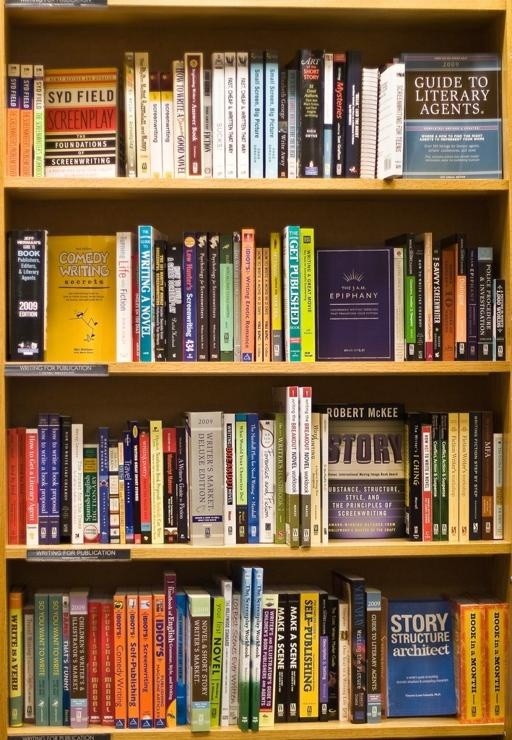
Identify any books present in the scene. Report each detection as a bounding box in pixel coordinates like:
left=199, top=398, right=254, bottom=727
left=7, top=225, right=506, bottom=361
left=9, top=565, right=511, bottom=732
left=6, top=47, right=503, bottom=179
left=7, top=385, right=503, bottom=548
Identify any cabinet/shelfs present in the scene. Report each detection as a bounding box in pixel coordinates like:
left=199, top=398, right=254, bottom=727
left=2, top=3, right=512, bottom=735
